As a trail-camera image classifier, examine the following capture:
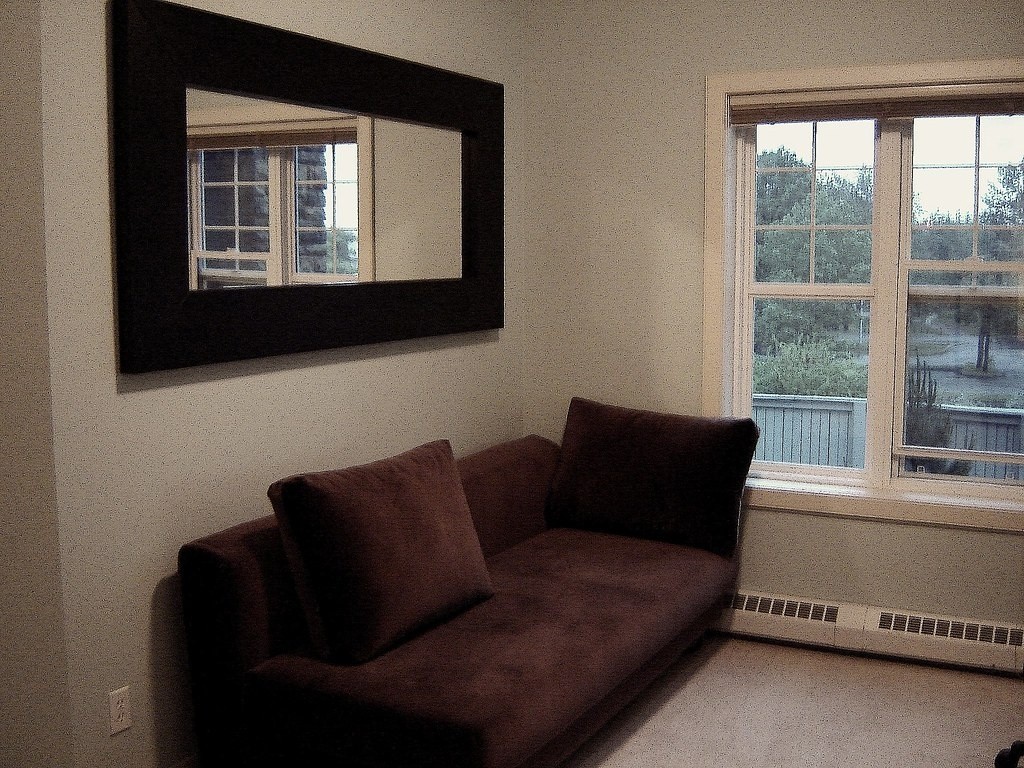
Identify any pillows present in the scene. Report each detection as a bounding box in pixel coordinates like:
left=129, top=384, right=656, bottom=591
left=268, top=438, right=495, bottom=664
left=545, top=397, right=760, bottom=557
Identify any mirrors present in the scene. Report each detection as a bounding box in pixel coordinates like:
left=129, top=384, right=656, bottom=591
left=106, top=1, right=504, bottom=375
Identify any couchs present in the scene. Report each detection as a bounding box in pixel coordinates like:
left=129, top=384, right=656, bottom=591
left=178, top=435, right=738, bottom=767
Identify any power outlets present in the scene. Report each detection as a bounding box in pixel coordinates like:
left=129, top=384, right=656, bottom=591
left=109, top=685, right=133, bottom=735
